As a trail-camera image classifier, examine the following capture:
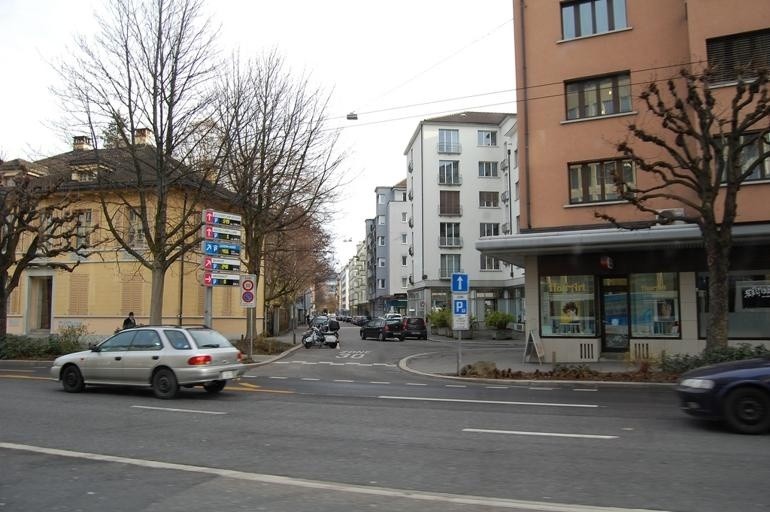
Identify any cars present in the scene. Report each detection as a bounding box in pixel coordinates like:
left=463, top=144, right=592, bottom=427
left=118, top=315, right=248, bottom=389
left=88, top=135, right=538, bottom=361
left=360, top=313, right=427, bottom=342
left=309, top=315, right=368, bottom=328
left=675, top=352, right=770, bottom=435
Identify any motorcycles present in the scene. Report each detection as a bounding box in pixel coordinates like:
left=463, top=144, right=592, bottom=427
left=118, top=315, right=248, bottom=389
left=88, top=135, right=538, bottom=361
left=302, top=323, right=340, bottom=350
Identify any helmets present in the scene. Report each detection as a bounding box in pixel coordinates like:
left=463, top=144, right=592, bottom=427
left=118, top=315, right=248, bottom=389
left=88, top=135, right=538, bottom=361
left=322, top=325, right=328, bottom=331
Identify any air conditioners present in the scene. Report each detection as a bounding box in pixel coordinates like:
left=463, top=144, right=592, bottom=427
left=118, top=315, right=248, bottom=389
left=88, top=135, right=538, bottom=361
left=655, top=207, right=685, bottom=225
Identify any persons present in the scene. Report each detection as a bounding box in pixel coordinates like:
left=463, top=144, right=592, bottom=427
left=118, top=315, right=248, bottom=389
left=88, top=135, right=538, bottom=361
left=658, top=299, right=675, bottom=320
left=563, top=302, right=580, bottom=333
left=304, top=309, right=310, bottom=324
left=123, top=312, right=136, bottom=328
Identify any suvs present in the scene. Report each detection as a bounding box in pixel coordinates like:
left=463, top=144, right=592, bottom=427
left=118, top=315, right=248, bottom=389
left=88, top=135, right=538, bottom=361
left=50, top=323, right=242, bottom=400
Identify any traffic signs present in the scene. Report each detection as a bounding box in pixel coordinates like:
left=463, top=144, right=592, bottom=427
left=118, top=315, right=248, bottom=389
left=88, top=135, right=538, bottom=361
left=202, top=209, right=242, bottom=288
